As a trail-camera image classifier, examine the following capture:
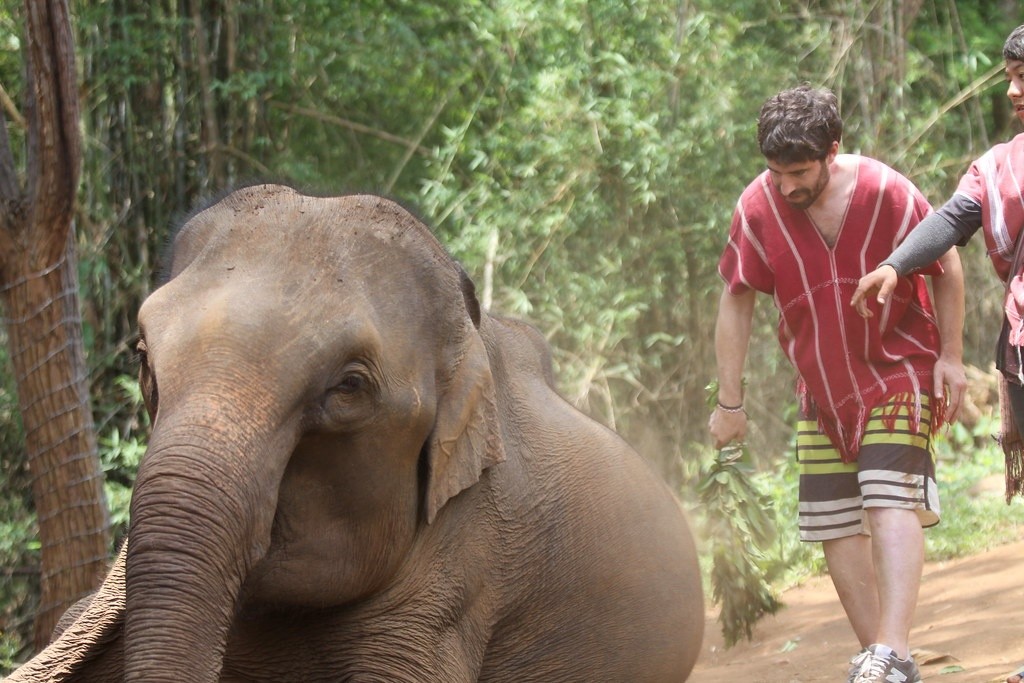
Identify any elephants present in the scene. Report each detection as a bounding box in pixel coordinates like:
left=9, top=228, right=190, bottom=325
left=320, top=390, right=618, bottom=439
left=0, top=174, right=704, bottom=683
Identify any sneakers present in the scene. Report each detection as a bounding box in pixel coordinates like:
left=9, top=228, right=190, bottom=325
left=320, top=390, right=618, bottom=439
left=846, top=644, right=923, bottom=683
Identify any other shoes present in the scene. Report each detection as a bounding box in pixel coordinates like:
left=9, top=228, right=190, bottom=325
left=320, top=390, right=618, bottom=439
left=1006, top=669, right=1024, bottom=683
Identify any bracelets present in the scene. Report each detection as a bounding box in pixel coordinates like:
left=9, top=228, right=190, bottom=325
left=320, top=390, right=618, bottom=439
left=716, top=400, right=743, bottom=413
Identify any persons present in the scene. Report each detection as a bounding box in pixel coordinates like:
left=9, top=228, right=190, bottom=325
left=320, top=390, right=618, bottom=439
left=708, top=83, right=966, bottom=682
left=851, top=24, right=1022, bottom=681
